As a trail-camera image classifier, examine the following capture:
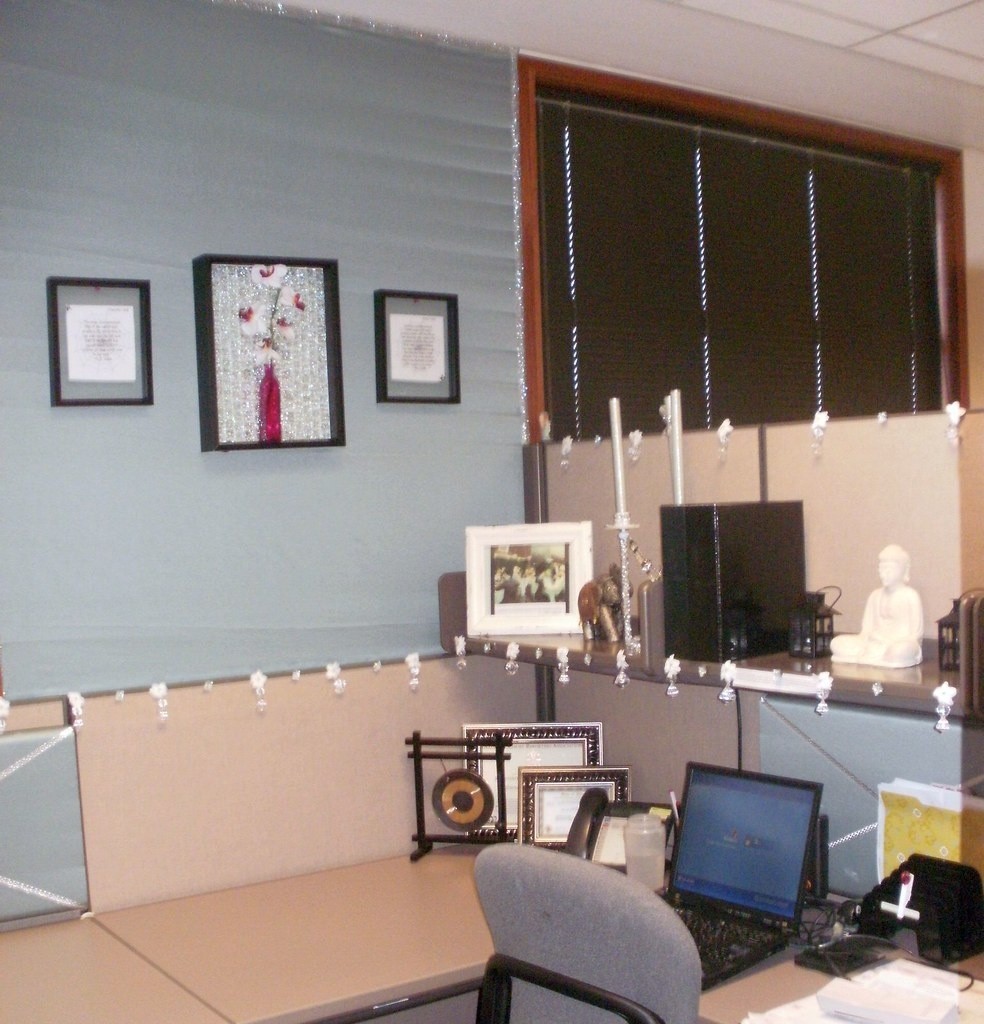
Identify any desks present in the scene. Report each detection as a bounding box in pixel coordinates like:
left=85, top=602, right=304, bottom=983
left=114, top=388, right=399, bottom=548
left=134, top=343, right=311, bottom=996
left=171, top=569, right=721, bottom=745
left=0, top=835, right=983, bottom=1024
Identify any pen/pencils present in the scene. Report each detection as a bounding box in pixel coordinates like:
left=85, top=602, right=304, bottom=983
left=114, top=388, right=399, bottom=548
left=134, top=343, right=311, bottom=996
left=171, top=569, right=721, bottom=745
left=669, top=790, right=680, bottom=827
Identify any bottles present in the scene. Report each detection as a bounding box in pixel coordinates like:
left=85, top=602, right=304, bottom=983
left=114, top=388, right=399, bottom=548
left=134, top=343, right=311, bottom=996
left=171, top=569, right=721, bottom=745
left=622, top=814, right=666, bottom=890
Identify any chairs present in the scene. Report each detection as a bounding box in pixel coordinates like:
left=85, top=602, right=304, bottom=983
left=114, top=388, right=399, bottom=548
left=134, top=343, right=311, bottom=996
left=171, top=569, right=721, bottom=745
left=473, top=839, right=701, bottom=1024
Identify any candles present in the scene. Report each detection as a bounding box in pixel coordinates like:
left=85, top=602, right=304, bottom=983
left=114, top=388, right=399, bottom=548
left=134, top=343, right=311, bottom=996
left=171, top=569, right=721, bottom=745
left=663, top=387, right=685, bottom=505
left=608, top=396, right=627, bottom=519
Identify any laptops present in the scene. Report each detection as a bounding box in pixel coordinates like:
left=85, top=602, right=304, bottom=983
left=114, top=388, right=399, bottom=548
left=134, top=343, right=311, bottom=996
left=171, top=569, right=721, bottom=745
left=657, top=760, right=825, bottom=993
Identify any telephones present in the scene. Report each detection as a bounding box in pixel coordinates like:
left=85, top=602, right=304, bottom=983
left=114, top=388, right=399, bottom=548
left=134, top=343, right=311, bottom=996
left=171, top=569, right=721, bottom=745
left=560, top=784, right=674, bottom=881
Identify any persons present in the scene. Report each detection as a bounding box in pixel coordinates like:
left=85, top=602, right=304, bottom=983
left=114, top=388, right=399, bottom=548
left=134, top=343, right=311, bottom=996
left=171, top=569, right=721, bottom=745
left=492, top=557, right=566, bottom=604
left=830, top=545, right=924, bottom=667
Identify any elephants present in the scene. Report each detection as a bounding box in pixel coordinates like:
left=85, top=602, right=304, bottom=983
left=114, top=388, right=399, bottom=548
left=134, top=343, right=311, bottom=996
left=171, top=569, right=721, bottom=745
left=577, top=561, right=634, bottom=644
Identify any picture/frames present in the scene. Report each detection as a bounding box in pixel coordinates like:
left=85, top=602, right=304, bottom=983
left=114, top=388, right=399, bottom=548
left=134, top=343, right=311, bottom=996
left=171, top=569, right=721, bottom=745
left=462, top=520, right=594, bottom=639
left=45, top=275, right=155, bottom=408
left=191, top=252, right=348, bottom=452
left=373, top=288, right=461, bottom=406
